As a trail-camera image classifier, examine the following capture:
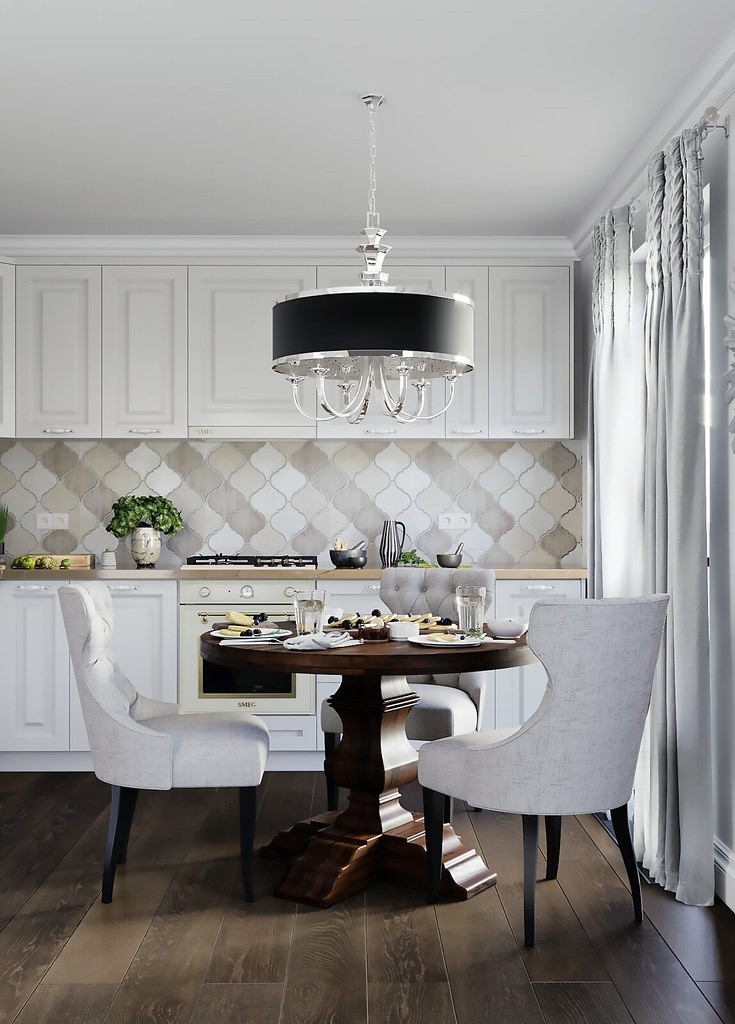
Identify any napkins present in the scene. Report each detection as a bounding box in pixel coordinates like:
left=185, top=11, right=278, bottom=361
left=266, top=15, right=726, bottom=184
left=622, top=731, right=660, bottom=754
left=219, top=638, right=284, bottom=646
left=485, top=640, right=516, bottom=644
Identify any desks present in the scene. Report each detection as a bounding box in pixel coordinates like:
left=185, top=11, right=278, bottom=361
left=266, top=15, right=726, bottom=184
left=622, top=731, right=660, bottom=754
left=200, top=621, right=540, bottom=909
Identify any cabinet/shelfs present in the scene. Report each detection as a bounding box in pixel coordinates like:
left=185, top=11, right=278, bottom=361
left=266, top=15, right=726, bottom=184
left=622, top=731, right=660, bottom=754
left=15, top=235, right=188, bottom=439
left=189, top=235, right=446, bottom=439
left=0, top=235, right=15, bottom=439
left=315, top=579, right=494, bottom=772
left=495, top=580, right=586, bottom=731
left=256, top=715, right=317, bottom=771
left=446, top=236, right=581, bottom=441
left=0, top=580, right=178, bottom=772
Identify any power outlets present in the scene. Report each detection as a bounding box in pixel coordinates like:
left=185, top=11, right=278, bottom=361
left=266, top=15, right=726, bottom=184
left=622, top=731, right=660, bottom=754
left=438, top=513, right=471, bottom=530
left=36, top=513, right=68, bottom=530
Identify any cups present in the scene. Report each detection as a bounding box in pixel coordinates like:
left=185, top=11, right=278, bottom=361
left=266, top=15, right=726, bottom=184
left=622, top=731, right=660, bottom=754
left=456, top=587, right=486, bottom=636
left=358, top=623, right=389, bottom=643
left=388, top=621, right=420, bottom=642
left=293, top=589, right=325, bottom=638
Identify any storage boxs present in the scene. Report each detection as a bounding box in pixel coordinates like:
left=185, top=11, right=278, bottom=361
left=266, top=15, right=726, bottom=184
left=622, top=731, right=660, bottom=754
left=28, top=554, right=95, bottom=567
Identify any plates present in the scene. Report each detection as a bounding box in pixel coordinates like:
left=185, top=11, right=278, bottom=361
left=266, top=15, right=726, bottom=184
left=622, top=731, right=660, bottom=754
left=407, top=633, right=493, bottom=647
left=210, top=627, right=292, bottom=640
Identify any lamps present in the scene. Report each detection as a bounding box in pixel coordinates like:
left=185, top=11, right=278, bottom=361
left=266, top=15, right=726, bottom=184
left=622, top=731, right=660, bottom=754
left=273, top=96, right=474, bottom=426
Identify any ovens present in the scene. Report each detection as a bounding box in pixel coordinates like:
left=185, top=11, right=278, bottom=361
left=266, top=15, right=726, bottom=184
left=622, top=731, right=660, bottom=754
left=178, top=602, right=317, bottom=716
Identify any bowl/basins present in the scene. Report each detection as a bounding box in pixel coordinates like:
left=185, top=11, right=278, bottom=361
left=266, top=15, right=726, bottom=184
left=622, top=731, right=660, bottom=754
left=487, top=619, right=529, bottom=638
left=348, top=557, right=367, bottom=568
left=436, top=554, right=462, bottom=568
left=329, top=549, right=367, bottom=569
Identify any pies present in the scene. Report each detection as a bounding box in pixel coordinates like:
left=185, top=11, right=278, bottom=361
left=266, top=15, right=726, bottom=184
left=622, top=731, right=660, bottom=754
left=329, top=614, right=460, bottom=630
left=220, top=612, right=255, bottom=636
left=428, top=633, right=458, bottom=641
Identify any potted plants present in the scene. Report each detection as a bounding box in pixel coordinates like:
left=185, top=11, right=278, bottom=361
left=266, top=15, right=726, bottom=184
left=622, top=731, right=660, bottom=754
left=0, top=500, right=8, bottom=555
left=106, top=495, right=186, bottom=571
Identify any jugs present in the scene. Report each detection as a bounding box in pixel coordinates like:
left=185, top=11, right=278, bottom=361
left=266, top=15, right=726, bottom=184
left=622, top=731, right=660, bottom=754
left=379, top=520, right=405, bottom=569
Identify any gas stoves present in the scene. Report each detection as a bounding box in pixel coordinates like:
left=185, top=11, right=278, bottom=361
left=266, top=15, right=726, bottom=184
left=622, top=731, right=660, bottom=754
left=180, top=552, right=318, bottom=605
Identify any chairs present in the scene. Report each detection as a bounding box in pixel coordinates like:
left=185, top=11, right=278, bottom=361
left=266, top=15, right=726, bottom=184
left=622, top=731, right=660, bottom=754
left=418, top=593, right=671, bottom=949
left=322, top=567, right=497, bottom=824
left=59, top=581, right=270, bottom=904
